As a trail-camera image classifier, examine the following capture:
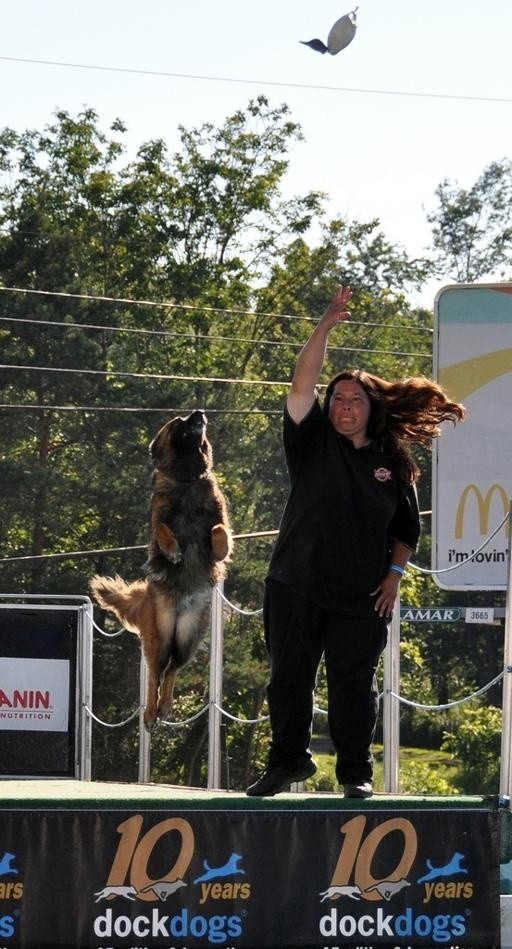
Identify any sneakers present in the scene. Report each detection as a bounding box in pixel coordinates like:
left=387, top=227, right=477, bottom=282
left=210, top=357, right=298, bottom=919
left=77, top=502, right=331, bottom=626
left=342, top=781, right=373, bottom=796
left=246, top=755, right=316, bottom=798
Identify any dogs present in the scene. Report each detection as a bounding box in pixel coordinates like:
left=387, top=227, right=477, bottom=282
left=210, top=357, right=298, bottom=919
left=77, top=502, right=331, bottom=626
left=86, top=409, right=235, bottom=734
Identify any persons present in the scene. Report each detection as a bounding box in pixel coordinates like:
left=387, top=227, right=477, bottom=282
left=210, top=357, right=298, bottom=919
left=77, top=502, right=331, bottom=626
left=244, top=281, right=467, bottom=798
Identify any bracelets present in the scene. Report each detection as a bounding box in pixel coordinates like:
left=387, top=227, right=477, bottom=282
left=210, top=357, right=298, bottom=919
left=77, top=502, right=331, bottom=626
left=388, top=561, right=404, bottom=576
